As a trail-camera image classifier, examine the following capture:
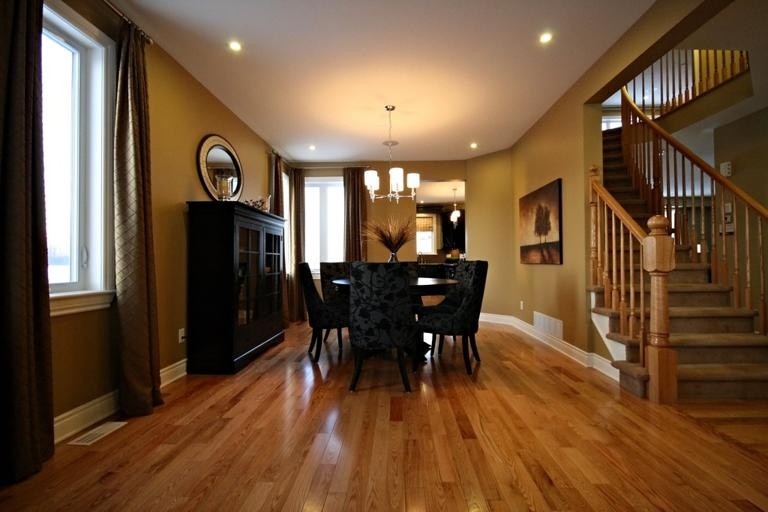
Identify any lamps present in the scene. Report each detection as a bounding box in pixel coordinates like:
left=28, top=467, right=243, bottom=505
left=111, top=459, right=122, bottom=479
left=450, top=189, right=461, bottom=230
left=416, top=231, right=438, bottom=263
left=364, top=106, right=420, bottom=204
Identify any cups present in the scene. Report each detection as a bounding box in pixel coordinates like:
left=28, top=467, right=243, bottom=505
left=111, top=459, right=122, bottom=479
left=215, top=174, right=233, bottom=201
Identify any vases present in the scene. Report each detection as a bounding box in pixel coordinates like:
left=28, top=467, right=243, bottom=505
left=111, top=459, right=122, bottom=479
left=388, top=253, right=399, bottom=263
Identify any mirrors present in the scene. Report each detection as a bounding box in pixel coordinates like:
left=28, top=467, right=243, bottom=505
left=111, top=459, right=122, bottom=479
left=197, top=134, right=244, bottom=202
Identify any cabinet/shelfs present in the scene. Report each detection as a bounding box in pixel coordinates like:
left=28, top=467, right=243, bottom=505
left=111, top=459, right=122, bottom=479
left=186, top=201, right=286, bottom=374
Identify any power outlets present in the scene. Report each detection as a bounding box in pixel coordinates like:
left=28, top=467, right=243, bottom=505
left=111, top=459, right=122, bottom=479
left=179, top=328, right=185, bottom=344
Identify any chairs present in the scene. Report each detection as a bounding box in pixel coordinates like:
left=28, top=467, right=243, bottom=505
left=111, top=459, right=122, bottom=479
left=300, top=261, right=488, bottom=392
